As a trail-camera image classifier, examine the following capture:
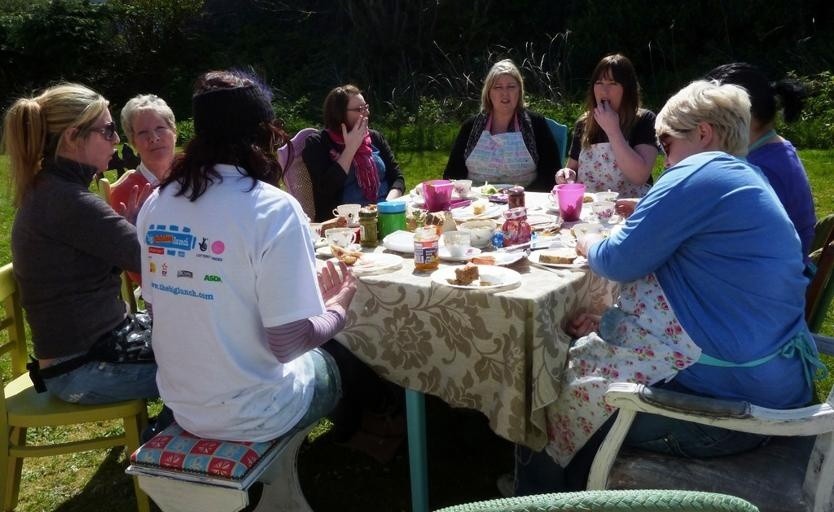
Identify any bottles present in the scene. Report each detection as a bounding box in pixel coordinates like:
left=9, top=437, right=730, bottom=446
left=508, top=184, right=525, bottom=211
left=413, top=226, right=439, bottom=274
left=378, top=201, right=407, bottom=243
left=359, top=209, right=378, bottom=246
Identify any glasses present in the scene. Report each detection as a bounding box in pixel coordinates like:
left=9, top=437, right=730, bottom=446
left=88, top=121, right=114, bottom=141
left=658, top=129, right=689, bottom=157
left=346, top=103, right=369, bottom=113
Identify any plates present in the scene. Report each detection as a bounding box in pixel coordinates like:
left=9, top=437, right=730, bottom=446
left=439, top=248, right=481, bottom=261
left=479, top=252, right=527, bottom=266
left=527, top=249, right=586, bottom=268
left=323, top=253, right=405, bottom=273
left=548, top=207, right=560, bottom=213
left=431, top=264, right=522, bottom=290
left=316, top=247, right=363, bottom=258
left=484, top=184, right=524, bottom=197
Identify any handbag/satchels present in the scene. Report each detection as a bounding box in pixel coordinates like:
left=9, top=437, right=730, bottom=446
left=30, top=312, right=154, bottom=394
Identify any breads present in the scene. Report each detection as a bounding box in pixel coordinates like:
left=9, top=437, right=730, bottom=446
left=539, top=249, right=576, bottom=266
left=455, top=262, right=480, bottom=285
left=330, top=243, right=360, bottom=265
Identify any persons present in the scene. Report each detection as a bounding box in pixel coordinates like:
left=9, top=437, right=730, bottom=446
left=496, top=80, right=829, bottom=496
left=554, top=54, right=660, bottom=199
left=6, top=84, right=174, bottom=444
left=706, top=63, right=817, bottom=268
left=136, top=71, right=365, bottom=439
left=444, top=60, right=562, bottom=193
left=301, top=85, right=406, bottom=222
left=109, top=93, right=179, bottom=288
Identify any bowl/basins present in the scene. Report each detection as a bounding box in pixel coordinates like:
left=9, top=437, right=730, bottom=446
left=459, top=221, right=496, bottom=249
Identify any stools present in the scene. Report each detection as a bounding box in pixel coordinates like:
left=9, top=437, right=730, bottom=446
left=123, top=420, right=321, bottom=512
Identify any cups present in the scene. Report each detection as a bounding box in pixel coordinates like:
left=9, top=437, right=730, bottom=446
left=422, top=180, right=454, bottom=212
left=325, top=229, right=356, bottom=247
left=596, top=192, right=619, bottom=201
left=444, top=231, right=470, bottom=256
left=548, top=189, right=560, bottom=207
left=591, top=201, right=617, bottom=223
left=553, top=184, right=586, bottom=222
left=453, top=179, right=473, bottom=198
left=332, top=204, right=361, bottom=227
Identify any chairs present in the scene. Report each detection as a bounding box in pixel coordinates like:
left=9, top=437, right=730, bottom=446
left=277, top=129, right=320, bottom=222
left=424, top=492, right=757, bottom=512
left=585, top=331, right=834, bottom=512
left=97, top=171, right=151, bottom=314
left=544, top=116, right=568, bottom=170
left=803, top=212, right=834, bottom=333
left=0, top=261, right=150, bottom=512
